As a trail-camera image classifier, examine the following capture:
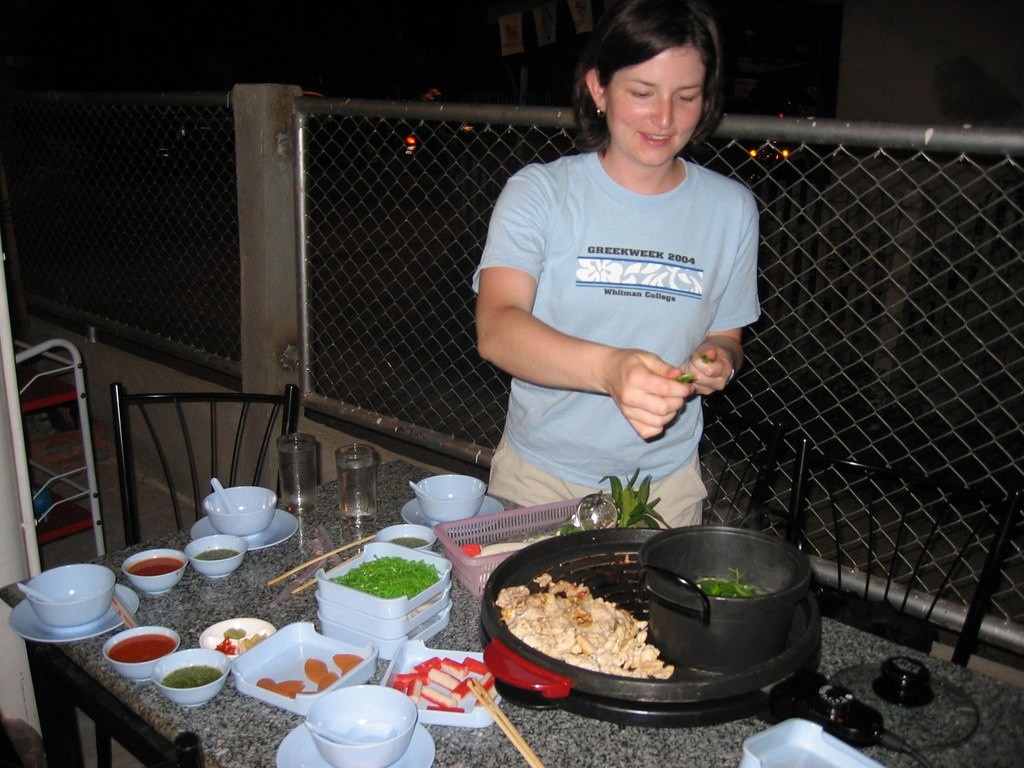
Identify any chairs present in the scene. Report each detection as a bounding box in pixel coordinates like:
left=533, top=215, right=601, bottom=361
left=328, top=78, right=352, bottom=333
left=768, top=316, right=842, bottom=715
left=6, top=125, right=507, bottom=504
left=786, top=440, right=1023, bottom=668
left=697, top=409, right=786, bottom=531
left=96, top=381, right=300, bottom=768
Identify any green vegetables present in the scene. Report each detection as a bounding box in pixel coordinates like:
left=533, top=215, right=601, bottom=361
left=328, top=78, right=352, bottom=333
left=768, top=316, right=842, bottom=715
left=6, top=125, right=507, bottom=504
left=701, top=568, right=758, bottom=598
left=328, top=556, right=440, bottom=600
left=558, top=468, right=671, bottom=533
left=677, top=353, right=709, bottom=384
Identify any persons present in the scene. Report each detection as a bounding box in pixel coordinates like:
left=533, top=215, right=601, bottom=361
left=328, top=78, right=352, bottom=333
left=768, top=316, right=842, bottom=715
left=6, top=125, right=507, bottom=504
left=470, top=0, right=762, bottom=535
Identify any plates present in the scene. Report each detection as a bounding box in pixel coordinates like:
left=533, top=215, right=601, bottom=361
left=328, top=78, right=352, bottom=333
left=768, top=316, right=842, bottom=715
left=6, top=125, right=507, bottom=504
left=315, top=541, right=453, bottom=660
left=276, top=722, right=435, bottom=768
left=400, top=496, right=503, bottom=532
left=379, top=636, right=502, bottom=728
left=738, top=717, right=886, bottom=768
left=231, top=621, right=379, bottom=717
left=191, top=509, right=298, bottom=550
left=200, top=617, right=277, bottom=658
left=8, top=584, right=139, bottom=642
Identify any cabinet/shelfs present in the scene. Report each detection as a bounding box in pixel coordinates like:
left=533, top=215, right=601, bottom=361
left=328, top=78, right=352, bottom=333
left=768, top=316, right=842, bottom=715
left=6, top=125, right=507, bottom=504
left=0, top=246, right=106, bottom=578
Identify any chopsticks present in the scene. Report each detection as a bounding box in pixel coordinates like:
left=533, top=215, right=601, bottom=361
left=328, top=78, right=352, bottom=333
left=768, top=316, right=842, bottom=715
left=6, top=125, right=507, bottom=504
left=466, top=677, right=546, bottom=768
left=268, top=534, right=377, bottom=594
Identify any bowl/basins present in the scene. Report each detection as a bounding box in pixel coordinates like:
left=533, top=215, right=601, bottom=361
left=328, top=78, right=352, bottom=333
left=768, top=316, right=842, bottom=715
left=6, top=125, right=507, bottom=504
left=26, top=563, right=116, bottom=628
left=184, top=535, right=248, bottom=578
left=375, top=523, right=437, bottom=551
left=152, top=648, right=231, bottom=707
left=103, top=626, right=181, bottom=681
left=121, top=549, right=189, bottom=594
left=307, top=684, right=418, bottom=768
left=414, top=474, right=486, bottom=524
left=203, top=486, right=278, bottom=536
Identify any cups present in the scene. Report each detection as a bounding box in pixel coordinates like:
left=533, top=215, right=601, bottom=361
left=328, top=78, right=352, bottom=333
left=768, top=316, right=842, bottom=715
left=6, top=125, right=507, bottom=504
left=335, top=444, right=377, bottom=524
left=276, top=432, right=316, bottom=515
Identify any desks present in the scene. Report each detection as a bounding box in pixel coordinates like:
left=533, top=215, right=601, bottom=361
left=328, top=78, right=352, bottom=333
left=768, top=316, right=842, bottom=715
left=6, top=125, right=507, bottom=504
left=0, top=459, right=1024, bottom=768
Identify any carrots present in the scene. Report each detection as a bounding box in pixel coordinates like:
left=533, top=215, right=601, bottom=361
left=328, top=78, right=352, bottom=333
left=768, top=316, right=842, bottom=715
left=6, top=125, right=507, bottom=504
left=257, top=654, right=364, bottom=699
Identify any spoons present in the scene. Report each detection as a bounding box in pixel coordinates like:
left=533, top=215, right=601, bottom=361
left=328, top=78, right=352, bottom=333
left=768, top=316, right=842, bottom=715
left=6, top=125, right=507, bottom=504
left=18, top=583, right=91, bottom=604
left=306, top=722, right=399, bottom=745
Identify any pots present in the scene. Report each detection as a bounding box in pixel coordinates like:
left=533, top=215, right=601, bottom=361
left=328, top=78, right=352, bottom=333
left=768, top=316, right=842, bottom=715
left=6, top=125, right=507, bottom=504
left=638, top=509, right=813, bottom=673
left=479, top=525, right=883, bottom=741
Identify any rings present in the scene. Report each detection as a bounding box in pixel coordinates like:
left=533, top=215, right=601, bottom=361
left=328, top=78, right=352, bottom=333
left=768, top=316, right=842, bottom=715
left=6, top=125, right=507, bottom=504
left=727, top=369, right=736, bottom=384
